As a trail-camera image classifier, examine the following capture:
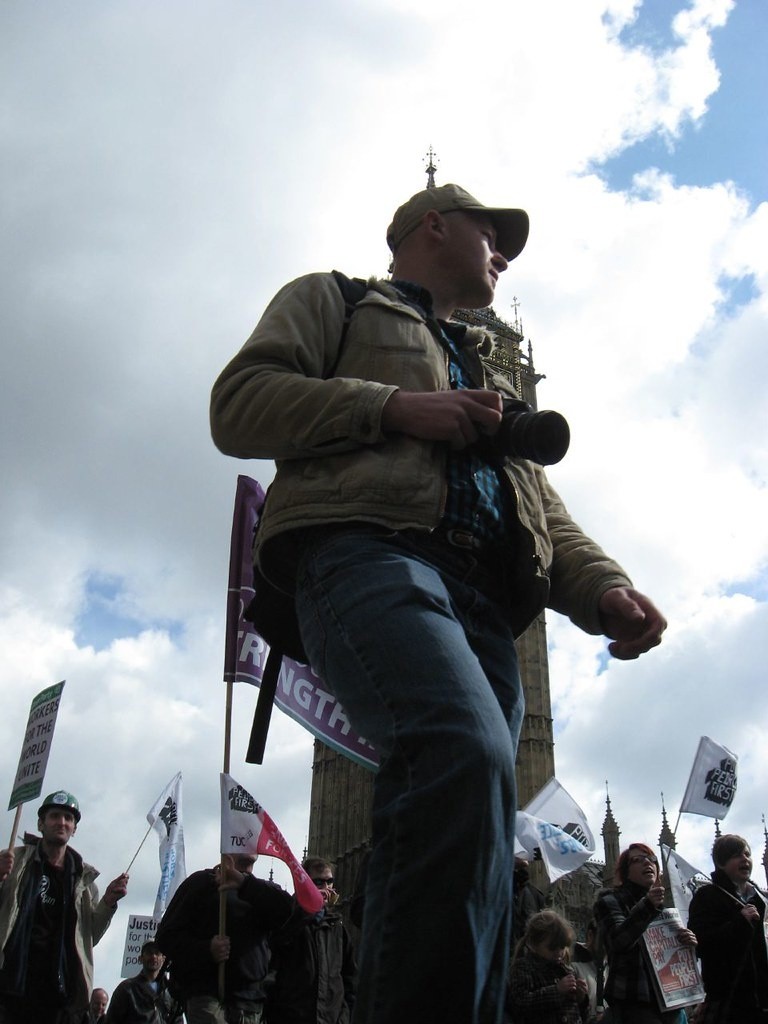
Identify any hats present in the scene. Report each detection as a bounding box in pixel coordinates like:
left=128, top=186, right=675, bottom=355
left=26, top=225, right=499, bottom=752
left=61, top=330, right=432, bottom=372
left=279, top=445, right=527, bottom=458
left=386, top=183, right=528, bottom=272
left=141, top=938, right=156, bottom=957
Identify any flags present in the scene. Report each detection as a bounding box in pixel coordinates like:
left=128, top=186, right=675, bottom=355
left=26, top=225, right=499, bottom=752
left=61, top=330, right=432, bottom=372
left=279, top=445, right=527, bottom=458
left=512, top=776, right=594, bottom=886
left=680, top=732, right=742, bottom=824
left=146, top=771, right=188, bottom=925
left=221, top=773, right=325, bottom=916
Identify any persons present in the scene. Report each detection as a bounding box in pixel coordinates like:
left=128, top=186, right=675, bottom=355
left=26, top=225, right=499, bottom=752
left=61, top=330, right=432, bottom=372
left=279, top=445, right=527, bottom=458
left=209, top=183, right=668, bottom=1024
left=0, top=789, right=768, bottom=1024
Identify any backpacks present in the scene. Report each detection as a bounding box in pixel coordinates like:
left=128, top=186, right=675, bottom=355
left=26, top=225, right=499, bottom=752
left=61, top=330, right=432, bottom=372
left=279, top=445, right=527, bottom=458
left=241, top=267, right=370, bottom=664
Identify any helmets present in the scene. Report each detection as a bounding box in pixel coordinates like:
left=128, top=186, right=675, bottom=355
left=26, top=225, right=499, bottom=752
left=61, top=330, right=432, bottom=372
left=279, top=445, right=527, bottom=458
left=38, top=790, right=82, bottom=824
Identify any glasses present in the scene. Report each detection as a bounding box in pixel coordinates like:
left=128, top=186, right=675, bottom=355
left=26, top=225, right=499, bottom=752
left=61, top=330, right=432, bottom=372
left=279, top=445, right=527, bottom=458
left=312, top=877, right=335, bottom=886
left=626, top=854, right=658, bottom=868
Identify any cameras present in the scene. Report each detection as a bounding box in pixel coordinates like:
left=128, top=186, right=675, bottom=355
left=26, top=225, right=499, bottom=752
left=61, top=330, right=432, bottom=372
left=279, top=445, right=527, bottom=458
left=489, top=398, right=570, bottom=466
